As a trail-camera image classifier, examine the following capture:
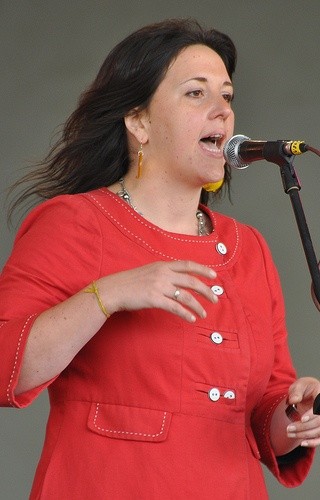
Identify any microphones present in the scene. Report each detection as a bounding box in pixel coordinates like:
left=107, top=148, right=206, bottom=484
left=223, top=134, right=308, bottom=169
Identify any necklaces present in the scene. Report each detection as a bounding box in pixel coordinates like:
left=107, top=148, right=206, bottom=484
left=116, top=178, right=208, bottom=237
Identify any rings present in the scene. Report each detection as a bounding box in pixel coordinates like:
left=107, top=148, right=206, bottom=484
left=1, top=20, right=320, bottom=500
left=175, top=288, right=180, bottom=301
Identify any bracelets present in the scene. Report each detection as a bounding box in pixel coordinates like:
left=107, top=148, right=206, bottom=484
left=83, top=281, right=110, bottom=319
left=285, top=397, right=300, bottom=422
